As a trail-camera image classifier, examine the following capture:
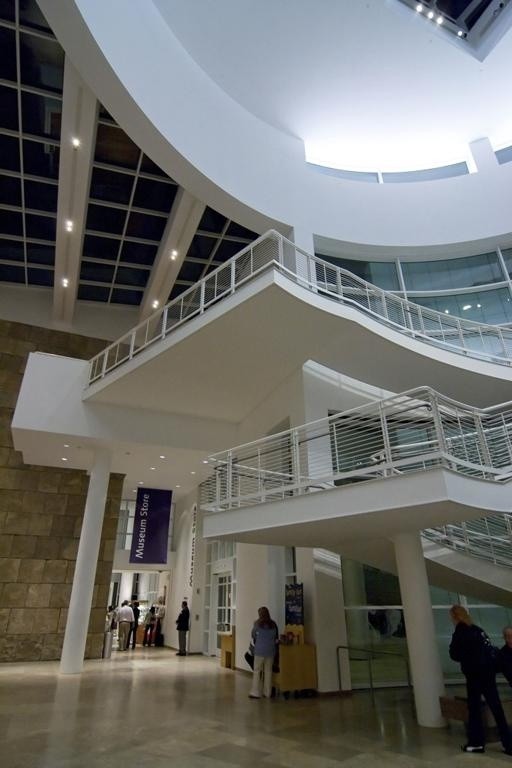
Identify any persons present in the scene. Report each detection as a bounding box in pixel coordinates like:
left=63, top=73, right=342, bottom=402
left=499, top=626, right=512, bottom=685
left=106, top=600, right=140, bottom=651
left=248, top=606, right=280, bottom=699
left=175, top=601, right=190, bottom=656
left=449, top=606, right=511, bottom=756
left=142, top=606, right=156, bottom=647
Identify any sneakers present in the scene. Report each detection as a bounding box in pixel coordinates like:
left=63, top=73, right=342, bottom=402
left=176, top=652, right=186, bottom=656
left=248, top=694, right=265, bottom=698
left=463, top=745, right=485, bottom=753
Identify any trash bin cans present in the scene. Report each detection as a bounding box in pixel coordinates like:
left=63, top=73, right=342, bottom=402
left=154, top=633, right=164, bottom=648
